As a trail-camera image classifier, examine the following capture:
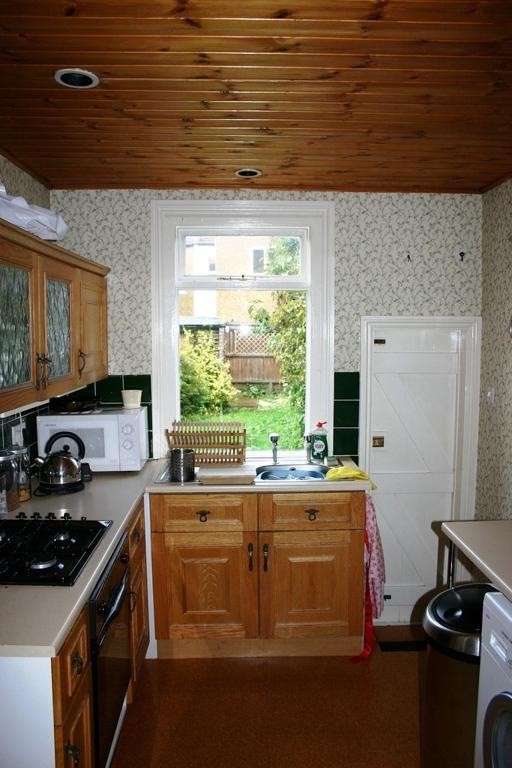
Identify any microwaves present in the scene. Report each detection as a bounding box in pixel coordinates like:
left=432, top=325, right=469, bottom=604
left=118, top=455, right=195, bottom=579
left=36, top=407, right=150, bottom=473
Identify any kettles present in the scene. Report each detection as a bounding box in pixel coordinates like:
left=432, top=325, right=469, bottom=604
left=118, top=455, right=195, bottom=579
left=33, top=432, right=93, bottom=496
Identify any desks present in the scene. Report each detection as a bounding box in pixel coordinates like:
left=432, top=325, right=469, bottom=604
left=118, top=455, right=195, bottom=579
left=430, top=518, right=512, bottom=603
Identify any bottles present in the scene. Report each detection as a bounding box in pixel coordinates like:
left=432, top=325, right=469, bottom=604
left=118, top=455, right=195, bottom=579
left=306, top=421, right=329, bottom=464
left=0, top=446, right=31, bottom=513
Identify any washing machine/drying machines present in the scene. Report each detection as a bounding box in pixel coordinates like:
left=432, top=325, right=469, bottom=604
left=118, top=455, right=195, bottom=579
left=472, top=591, right=512, bottom=768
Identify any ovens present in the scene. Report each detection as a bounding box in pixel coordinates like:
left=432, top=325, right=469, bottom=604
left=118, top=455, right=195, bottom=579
left=90, top=530, right=133, bottom=768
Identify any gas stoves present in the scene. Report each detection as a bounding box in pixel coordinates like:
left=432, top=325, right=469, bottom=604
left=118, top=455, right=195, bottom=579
left=0, top=512, right=114, bottom=586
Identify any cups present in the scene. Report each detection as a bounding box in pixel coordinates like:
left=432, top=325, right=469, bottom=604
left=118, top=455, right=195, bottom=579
left=121, top=390, right=142, bottom=409
left=171, top=448, right=195, bottom=482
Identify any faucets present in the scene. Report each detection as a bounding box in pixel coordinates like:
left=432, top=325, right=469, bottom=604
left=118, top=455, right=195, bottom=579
left=270, top=434, right=279, bottom=462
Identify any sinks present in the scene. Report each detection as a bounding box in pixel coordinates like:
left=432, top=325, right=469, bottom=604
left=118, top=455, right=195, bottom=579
left=254, top=462, right=333, bottom=482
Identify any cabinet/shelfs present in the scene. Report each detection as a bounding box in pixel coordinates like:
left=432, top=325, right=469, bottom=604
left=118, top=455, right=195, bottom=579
left=0, top=237, right=77, bottom=413
left=51, top=608, right=93, bottom=768
left=75, top=268, right=107, bottom=387
left=149, top=493, right=366, bottom=657
left=128, top=504, right=150, bottom=681
